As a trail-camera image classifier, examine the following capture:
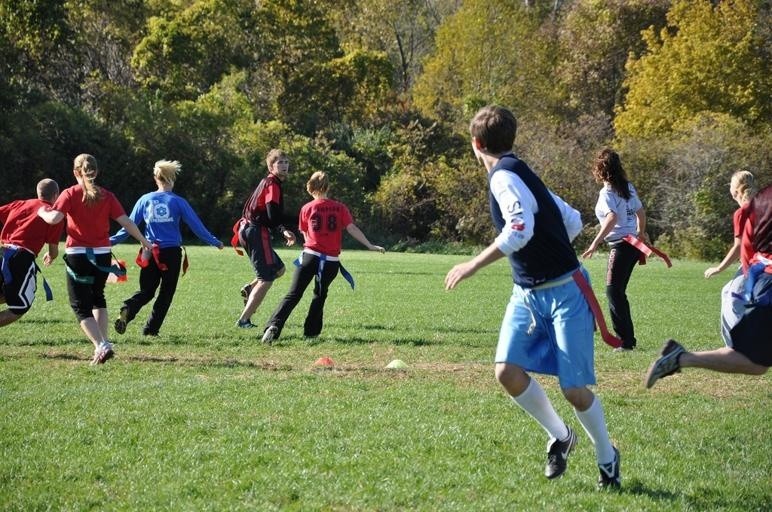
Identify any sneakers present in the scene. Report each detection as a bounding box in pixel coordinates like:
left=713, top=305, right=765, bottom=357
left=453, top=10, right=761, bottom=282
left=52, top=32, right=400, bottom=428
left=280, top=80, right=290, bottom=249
left=105, top=340, right=112, bottom=348
left=89, top=342, right=113, bottom=364
left=302, top=335, right=319, bottom=341
left=646, top=339, right=686, bottom=388
left=596, top=445, right=621, bottom=495
left=262, top=325, right=278, bottom=346
left=235, top=319, right=257, bottom=329
left=545, top=425, right=577, bottom=479
left=114, top=306, right=129, bottom=334
left=142, top=328, right=160, bottom=336
left=240, top=283, right=256, bottom=314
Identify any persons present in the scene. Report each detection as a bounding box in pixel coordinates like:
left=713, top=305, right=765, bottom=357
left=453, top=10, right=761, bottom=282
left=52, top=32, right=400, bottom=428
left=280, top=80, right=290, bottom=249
left=108, top=159, right=223, bottom=337
left=444, top=105, right=622, bottom=491
left=703, top=170, right=758, bottom=343
left=644, top=184, right=772, bottom=389
left=260, top=170, right=386, bottom=346
left=235, top=149, right=297, bottom=330
left=37, top=152, right=153, bottom=366
left=0, top=177, right=60, bottom=325
left=581, top=149, right=673, bottom=353
